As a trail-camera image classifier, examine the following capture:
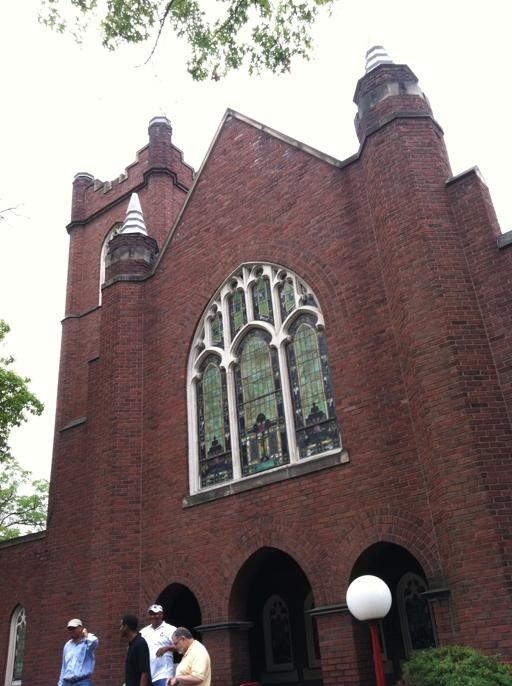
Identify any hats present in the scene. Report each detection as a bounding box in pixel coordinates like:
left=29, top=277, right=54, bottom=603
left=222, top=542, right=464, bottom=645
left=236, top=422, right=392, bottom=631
left=65, top=618, right=83, bottom=630
left=146, top=604, right=164, bottom=614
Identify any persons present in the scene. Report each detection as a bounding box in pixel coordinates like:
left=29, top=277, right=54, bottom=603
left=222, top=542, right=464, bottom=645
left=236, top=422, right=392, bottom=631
left=165, top=627, right=212, bottom=686
left=56, top=618, right=99, bottom=686
left=119, top=614, right=153, bottom=686
left=137, top=604, right=177, bottom=686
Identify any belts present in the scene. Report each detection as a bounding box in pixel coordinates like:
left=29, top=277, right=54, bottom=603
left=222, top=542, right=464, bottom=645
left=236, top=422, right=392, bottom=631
left=62, top=673, right=91, bottom=684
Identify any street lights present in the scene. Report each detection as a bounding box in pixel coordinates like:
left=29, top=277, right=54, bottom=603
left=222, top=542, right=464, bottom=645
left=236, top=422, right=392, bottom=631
left=346, top=574, right=392, bottom=686
